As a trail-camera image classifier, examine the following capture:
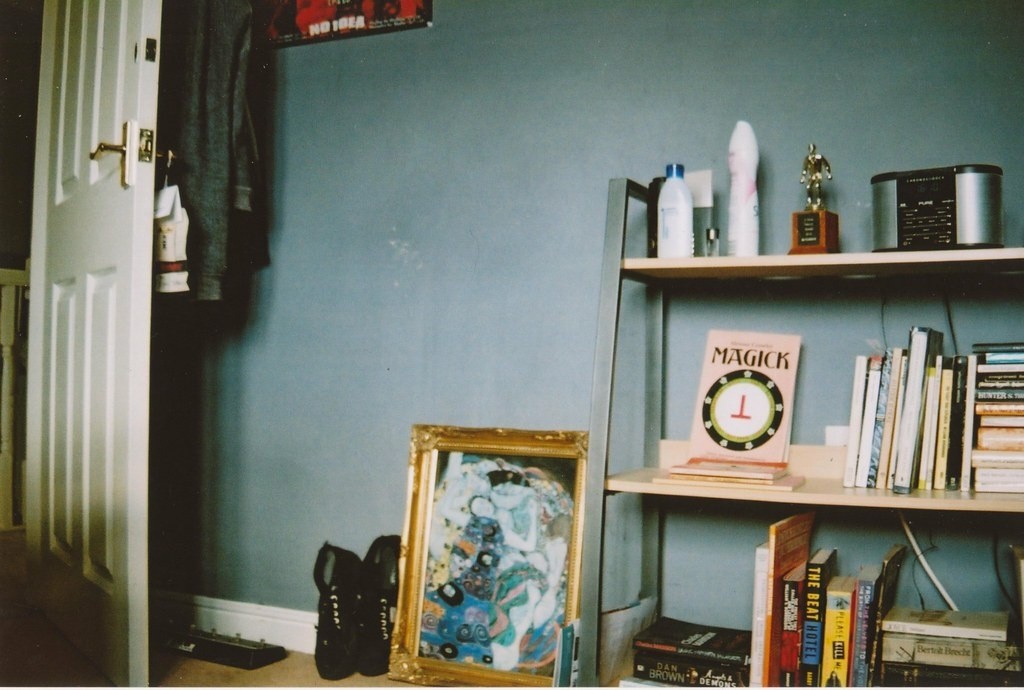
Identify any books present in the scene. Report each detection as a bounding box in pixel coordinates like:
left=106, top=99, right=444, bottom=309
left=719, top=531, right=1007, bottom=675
left=619, top=514, right=1024, bottom=690
left=843, top=326, right=1024, bottom=495
left=653, top=457, right=806, bottom=492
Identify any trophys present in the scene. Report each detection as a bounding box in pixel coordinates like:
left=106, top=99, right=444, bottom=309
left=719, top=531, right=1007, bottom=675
left=788, top=145, right=839, bottom=253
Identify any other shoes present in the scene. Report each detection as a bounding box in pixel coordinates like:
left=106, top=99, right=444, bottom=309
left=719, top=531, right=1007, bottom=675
left=313, top=540, right=360, bottom=681
left=355, top=535, right=401, bottom=677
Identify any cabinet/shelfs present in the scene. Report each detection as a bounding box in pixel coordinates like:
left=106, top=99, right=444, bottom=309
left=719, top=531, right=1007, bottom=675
left=572, top=176, right=1023, bottom=688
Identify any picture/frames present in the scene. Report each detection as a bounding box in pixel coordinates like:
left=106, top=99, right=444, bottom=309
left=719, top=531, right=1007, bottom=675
left=387, top=424, right=589, bottom=688
